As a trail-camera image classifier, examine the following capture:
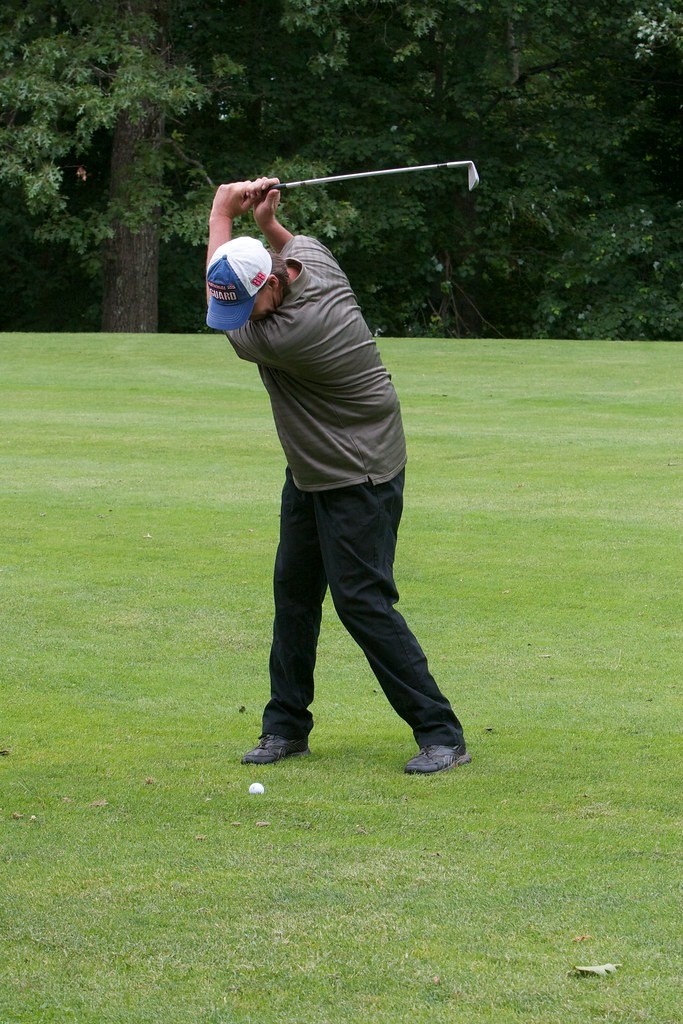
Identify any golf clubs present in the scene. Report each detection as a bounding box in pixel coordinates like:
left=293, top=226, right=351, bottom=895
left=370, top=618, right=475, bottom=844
left=269, top=160, right=481, bottom=191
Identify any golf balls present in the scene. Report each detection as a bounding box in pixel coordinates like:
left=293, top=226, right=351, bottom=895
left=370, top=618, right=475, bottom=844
left=249, top=782, right=265, bottom=796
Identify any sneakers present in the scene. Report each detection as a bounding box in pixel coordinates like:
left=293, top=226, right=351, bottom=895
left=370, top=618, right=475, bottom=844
left=241, top=734, right=308, bottom=764
left=405, top=745, right=471, bottom=774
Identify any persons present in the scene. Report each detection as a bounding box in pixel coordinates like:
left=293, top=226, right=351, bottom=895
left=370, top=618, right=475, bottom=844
left=205, top=177, right=472, bottom=774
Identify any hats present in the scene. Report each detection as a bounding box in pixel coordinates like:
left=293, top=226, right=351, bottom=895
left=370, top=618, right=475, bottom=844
left=206, top=236, right=273, bottom=330
